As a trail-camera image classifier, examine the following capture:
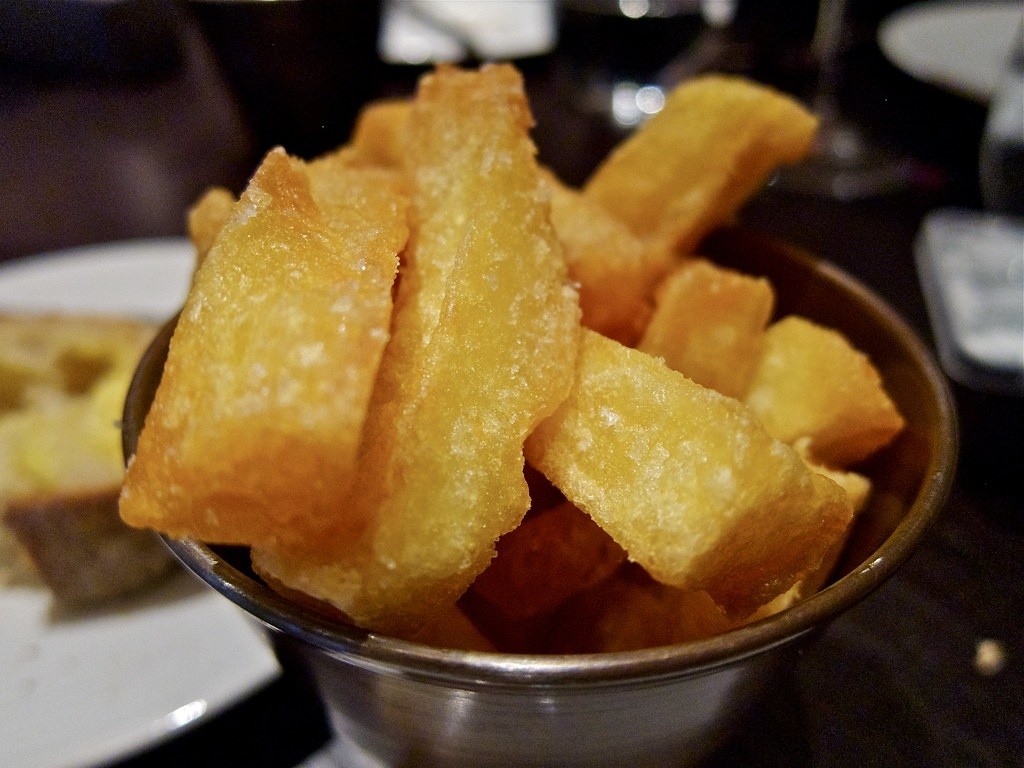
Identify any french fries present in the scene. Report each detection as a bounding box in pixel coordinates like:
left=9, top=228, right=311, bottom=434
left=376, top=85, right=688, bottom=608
left=119, top=64, right=908, bottom=656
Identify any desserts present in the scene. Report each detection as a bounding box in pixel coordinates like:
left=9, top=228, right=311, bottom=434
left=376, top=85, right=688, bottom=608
left=0, top=309, right=186, bottom=609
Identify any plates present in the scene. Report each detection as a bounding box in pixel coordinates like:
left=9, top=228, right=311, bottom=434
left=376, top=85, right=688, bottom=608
left=879, top=0, right=1024, bottom=103
left=0, top=238, right=302, bottom=768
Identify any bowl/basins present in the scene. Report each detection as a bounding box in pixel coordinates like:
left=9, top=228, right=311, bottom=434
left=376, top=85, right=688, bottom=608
left=121, top=219, right=952, bottom=768
